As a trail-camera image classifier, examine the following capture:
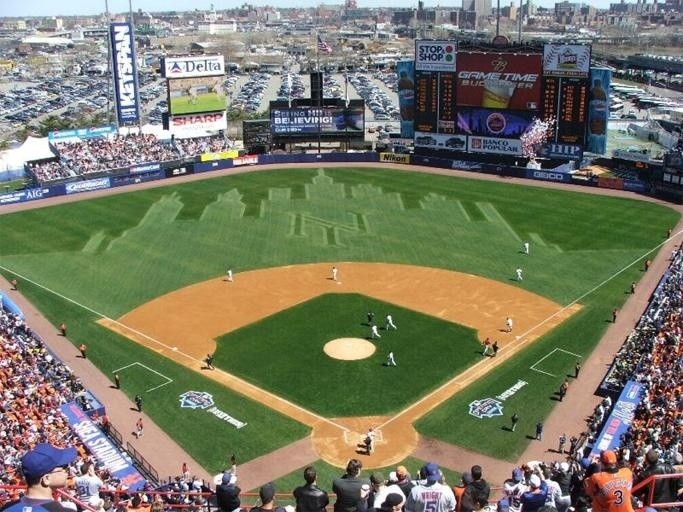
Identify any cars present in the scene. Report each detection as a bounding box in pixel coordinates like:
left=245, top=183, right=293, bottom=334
left=417, top=136, right=435, bottom=145
left=322, top=74, right=344, bottom=99
left=608, top=81, right=683, bottom=123
left=447, top=137, right=465, bottom=147
left=367, top=124, right=394, bottom=140
left=223, top=70, right=270, bottom=112
left=0, top=75, right=112, bottom=127
left=276, top=71, right=305, bottom=101
left=136, top=68, right=168, bottom=123
left=350, top=73, right=399, bottom=121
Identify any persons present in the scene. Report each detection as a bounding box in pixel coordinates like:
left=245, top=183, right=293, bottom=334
left=511, top=412, right=520, bottom=431
left=333, top=266, right=337, bottom=280
left=227, top=268, right=232, bottom=281
left=524, top=241, right=529, bottom=254
left=387, top=350, right=397, bottom=367
left=516, top=267, right=523, bottom=280
left=213, top=454, right=562, bottom=512
left=371, top=323, right=381, bottom=339
left=11, top=277, right=17, bottom=290
left=0, top=295, right=204, bottom=512
left=506, top=317, right=512, bottom=332
left=365, top=427, right=375, bottom=456
left=23, top=129, right=231, bottom=184
left=385, top=312, right=397, bottom=331
left=558, top=242, right=683, bottom=512
left=482, top=338, right=499, bottom=358
left=367, top=310, right=375, bottom=327
left=205, top=354, right=215, bottom=369
left=536, top=423, right=543, bottom=440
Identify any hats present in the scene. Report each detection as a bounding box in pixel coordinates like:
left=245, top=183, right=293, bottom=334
left=512, top=459, right=589, bottom=489
left=370, top=463, right=440, bottom=485
left=19, top=442, right=78, bottom=479
left=260, top=488, right=274, bottom=501
left=602, top=451, right=618, bottom=473
left=222, top=475, right=231, bottom=482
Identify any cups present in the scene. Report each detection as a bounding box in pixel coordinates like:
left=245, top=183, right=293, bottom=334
left=361, top=484, right=369, bottom=500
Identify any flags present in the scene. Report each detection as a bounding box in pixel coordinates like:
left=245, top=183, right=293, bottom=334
left=316, top=35, right=333, bottom=56
